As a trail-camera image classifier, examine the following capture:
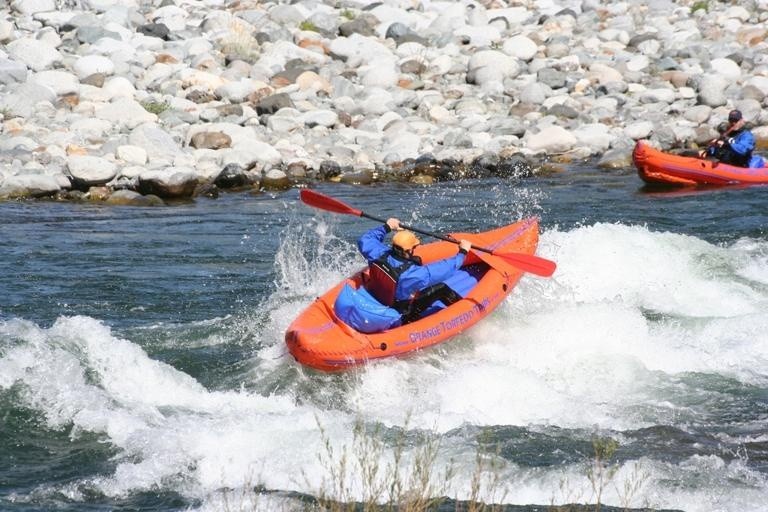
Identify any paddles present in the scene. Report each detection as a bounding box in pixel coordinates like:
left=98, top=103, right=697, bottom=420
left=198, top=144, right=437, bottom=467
left=701, top=118, right=746, bottom=156
left=301, top=189, right=557, bottom=277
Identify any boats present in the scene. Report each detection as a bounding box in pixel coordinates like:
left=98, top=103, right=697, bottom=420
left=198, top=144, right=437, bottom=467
left=283, top=213, right=541, bottom=372
left=630, top=139, right=766, bottom=190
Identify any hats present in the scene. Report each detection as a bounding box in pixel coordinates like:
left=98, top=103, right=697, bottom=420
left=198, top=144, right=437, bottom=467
left=717, top=109, right=742, bottom=133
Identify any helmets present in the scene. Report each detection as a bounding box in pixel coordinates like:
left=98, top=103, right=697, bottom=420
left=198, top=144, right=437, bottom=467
left=392, top=230, right=421, bottom=251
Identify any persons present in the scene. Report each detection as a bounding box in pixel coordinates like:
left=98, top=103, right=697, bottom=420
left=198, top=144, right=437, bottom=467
left=716, top=122, right=730, bottom=135
left=357, top=217, right=490, bottom=323
left=696, top=109, right=756, bottom=168
